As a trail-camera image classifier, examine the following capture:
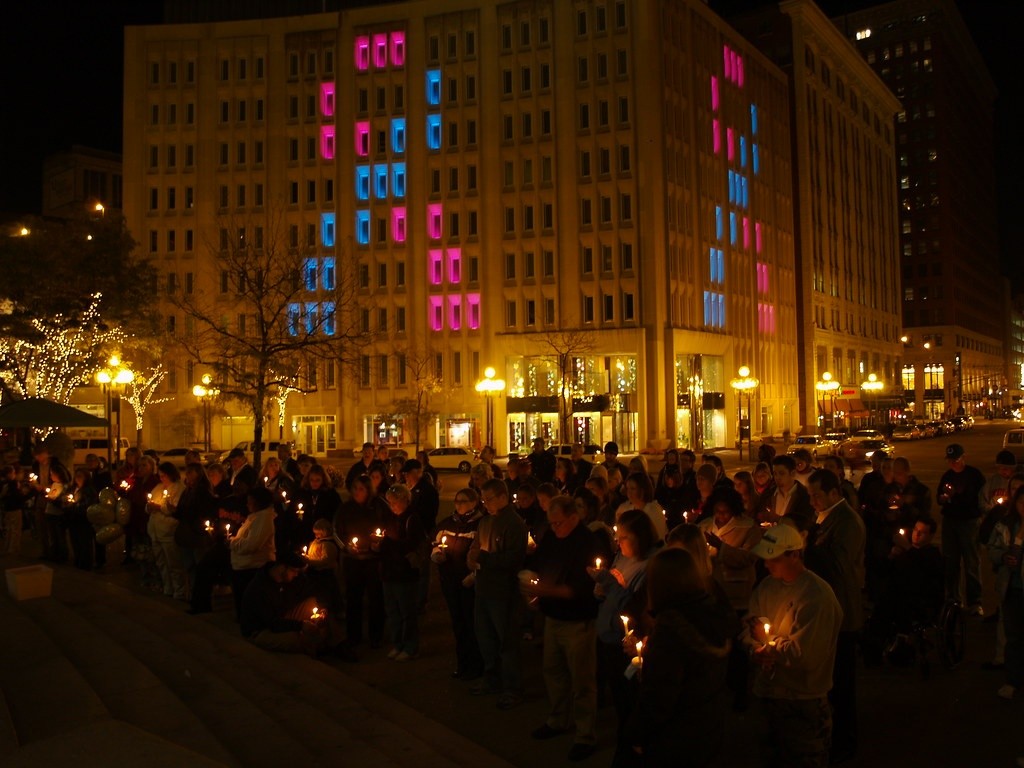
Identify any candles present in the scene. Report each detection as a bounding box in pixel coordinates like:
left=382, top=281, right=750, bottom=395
left=26, top=468, right=905, bottom=670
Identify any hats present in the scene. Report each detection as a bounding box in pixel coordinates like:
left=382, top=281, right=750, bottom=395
left=995, top=450, right=1017, bottom=465
left=750, top=525, right=806, bottom=560
left=603, top=442, right=618, bottom=456
left=228, top=448, right=244, bottom=459
left=291, top=454, right=317, bottom=464
left=398, top=459, right=423, bottom=473
left=944, top=444, right=965, bottom=460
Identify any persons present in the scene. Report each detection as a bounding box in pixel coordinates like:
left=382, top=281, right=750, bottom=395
left=431, top=437, right=1024, bottom=768
left=945, top=405, right=965, bottom=424
left=0, top=443, right=439, bottom=661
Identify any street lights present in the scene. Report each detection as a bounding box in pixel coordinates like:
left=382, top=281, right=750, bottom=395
left=730, top=364, right=760, bottom=460
left=95, top=352, right=134, bottom=474
left=474, top=366, right=506, bottom=448
left=192, top=373, right=221, bottom=452
left=863, top=373, right=883, bottom=420
left=815, top=371, right=840, bottom=434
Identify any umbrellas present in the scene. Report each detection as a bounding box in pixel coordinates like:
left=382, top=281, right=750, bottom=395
left=0, top=398, right=111, bottom=470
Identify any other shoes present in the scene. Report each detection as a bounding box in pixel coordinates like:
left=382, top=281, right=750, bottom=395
left=972, top=605, right=985, bottom=616
left=183, top=604, right=213, bottom=615
left=997, top=685, right=1019, bottom=699
left=470, top=681, right=502, bottom=696
left=387, top=647, right=421, bottom=662
left=981, top=661, right=1007, bottom=670
left=449, top=669, right=481, bottom=681
left=567, top=739, right=601, bottom=761
left=532, top=719, right=578, bottom=738
left=496, top=687, right=526, bottom=710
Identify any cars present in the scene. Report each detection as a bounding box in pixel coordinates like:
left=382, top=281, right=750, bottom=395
left=844, top=438, right=896, bottom=464
left=788, top=434, right=833, bottom=459
left=544, top=442, right=605, bottom=464
left=848, top=429, right=884, bottom=442
left=159, top=447, right=215, bottom=468
left=892, top=414, right=975, bottom=441
left=427, top=446, right=480, bottom=474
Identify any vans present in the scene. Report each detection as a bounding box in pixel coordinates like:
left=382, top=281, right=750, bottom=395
left=217, top=437, right=297, bottom=470
left=69, top=436, right=131, bottom=471
left=1003, top=429, right=1024, bottom=464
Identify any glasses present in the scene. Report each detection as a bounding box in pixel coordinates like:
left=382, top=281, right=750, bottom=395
left=389, top=486, right=398, bottom=493
left=479, top=494, right=499, bottom=503
left=453, top=499, right=470, bottom=504
left=547, top=515, right=569, bottom=527
